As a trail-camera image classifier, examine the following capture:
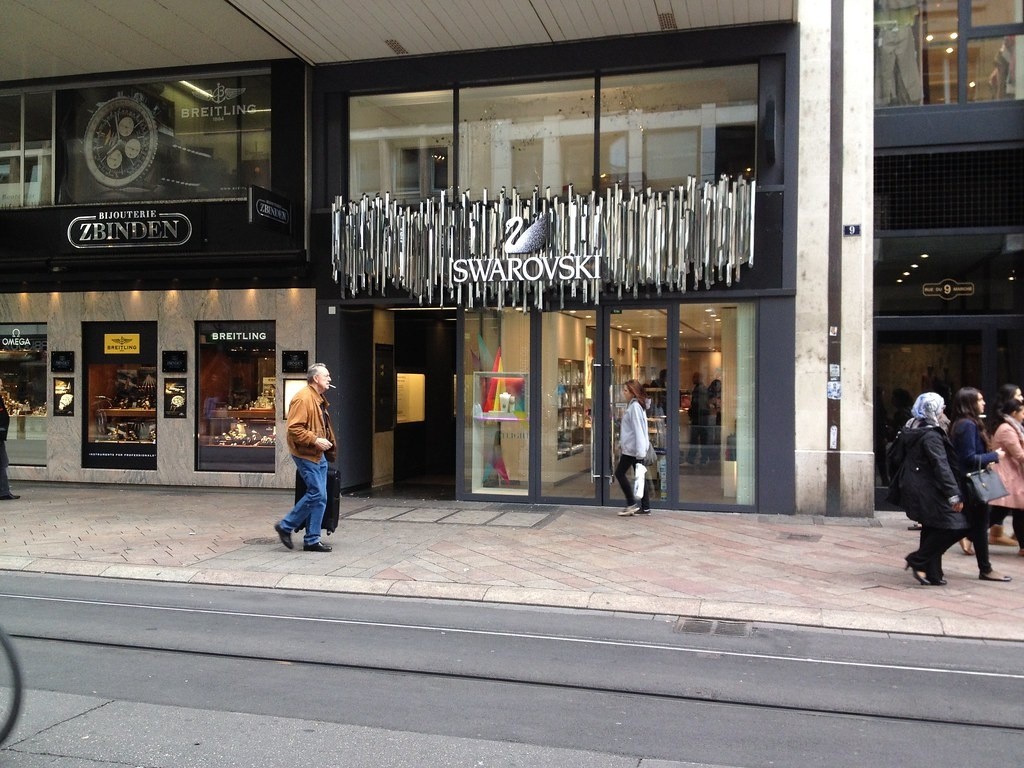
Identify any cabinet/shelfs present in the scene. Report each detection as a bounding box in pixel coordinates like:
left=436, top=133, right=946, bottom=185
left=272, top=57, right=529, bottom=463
left=500, top=310, right=659, bottom=487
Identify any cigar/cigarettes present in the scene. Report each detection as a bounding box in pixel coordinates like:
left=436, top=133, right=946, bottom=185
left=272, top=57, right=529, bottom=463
left=329, top=385, right=336, bottom=388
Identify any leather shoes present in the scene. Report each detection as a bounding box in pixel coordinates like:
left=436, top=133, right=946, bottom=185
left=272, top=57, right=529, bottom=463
left=303, top=542, right=332, bottom=552
left=0, top=494, right=19, bottom=500
left=275, top=524, right=294, bottom=549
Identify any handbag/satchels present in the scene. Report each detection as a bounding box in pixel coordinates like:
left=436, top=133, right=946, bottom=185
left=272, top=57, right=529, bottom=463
left=642, top=442, right=657, bottom=466
left=886, top=432, right=906, bottom=477
left=883, top=463, right=905, bottom=508
left=966, top=455, right=1009, bottom=504
left=632, top=463, right=648, bottom=501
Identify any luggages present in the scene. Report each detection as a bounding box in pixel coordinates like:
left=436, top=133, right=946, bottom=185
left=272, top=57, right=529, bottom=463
left=294, top=466, right=341, bottom=536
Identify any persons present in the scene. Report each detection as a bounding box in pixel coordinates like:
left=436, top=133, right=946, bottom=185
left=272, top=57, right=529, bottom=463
left=274, top=363, right=337, bottom=552
left=614, top=379, right=651, bottom=516
left=707, top=379, right=721, bottom=446
left=874, top=0, right=923, bottom=106
left=649, top=369, right=667, bottom=423
left=0, top=379, right=20, bottom=500
left=679, top=372, right=710, bottom=466
left=900, top=383, right=1024, bottom=585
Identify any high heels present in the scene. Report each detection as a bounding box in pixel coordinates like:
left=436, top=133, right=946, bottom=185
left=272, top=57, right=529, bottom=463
left=921, top=577, right=947, bottom=585
left=905, top=562, right=931, bottom=585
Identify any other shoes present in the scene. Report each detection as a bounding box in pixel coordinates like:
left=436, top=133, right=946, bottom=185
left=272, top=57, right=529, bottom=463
left=908, top=522, right=922, bottom=530
left=959, top=539, right=974, bottom=555
left=618, top=503, right=640, bottom=515
left=979, top=573, right=1012, bottom=581
left=634, top=507, right=652, bottom=516
left=1018, top=550, right=1024, bottom=555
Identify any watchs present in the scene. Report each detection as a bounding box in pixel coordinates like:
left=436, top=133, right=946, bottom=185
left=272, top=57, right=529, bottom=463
left=171, top=396, right=186, bottom=412
left=58, top=394, right=74, bottom=411
left=60, top=90, right=212, bottom=192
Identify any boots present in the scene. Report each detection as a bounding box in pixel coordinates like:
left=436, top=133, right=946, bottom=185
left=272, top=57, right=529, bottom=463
left=988, top=524, right=1019, bottom=546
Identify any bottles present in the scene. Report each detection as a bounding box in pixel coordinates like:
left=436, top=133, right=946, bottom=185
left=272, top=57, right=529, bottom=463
left=264, top=389, right=268, bottom=396
left=16, top=415, right=26, bottom=440
left=270, top=388, right=274, bottom=397
left=499, top=392, right=515, bottom=412
